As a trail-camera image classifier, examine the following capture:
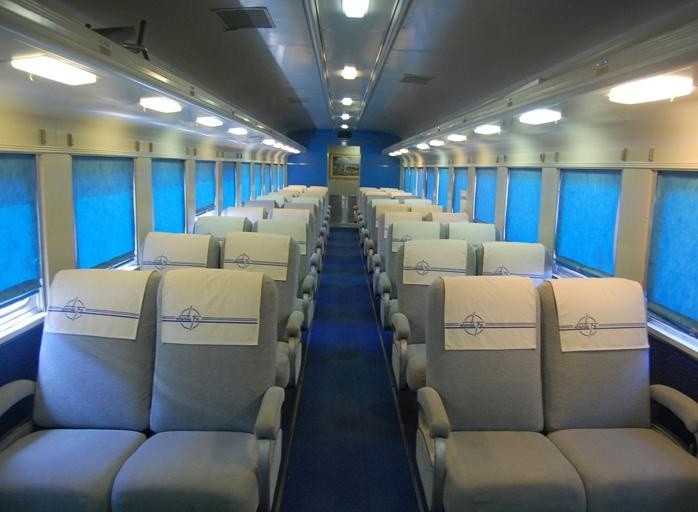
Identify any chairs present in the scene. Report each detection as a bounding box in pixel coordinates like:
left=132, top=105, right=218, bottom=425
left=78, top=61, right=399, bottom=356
left=352, top=185, right=697, bottom=505
left=2, top=182, right=332, bottom=509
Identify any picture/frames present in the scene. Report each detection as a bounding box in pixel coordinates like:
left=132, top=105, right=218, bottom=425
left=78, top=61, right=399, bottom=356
left=328, top=151, right=361, bottom=180
